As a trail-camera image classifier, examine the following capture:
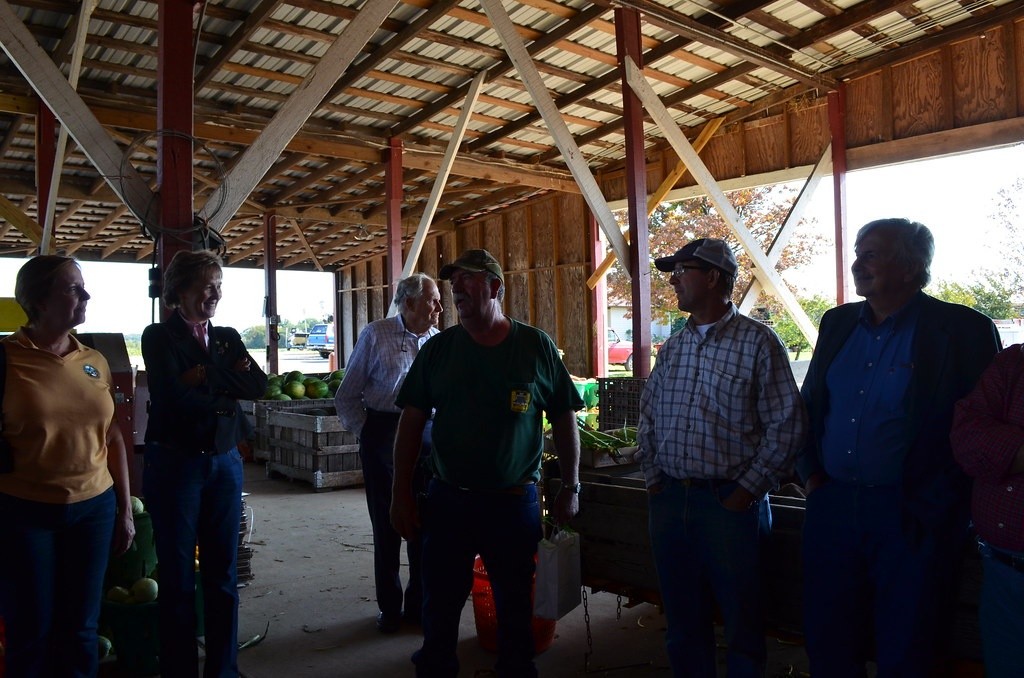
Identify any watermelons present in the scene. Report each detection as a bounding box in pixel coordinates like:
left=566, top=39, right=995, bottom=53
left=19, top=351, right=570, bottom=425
left=260, top=369, right=345, bottom=415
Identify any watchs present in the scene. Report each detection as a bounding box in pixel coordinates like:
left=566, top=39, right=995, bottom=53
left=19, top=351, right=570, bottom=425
left=746, top=499, right=759, bottom=510
left=560, top=482, right=581, bottom=493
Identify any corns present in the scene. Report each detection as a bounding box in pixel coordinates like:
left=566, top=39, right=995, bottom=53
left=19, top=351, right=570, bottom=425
left=579, top=426, right=638, bottom=451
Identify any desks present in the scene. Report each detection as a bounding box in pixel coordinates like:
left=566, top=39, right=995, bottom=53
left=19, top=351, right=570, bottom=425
left=549, top=455, right=810, bottom=636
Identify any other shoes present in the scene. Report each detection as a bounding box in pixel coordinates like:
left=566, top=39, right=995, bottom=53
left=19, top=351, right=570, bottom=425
left=376, top=610, right=400, bottom=634
left=404, top=608, right=422, bottom=626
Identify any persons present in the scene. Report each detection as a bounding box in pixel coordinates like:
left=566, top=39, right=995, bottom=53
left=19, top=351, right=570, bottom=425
left=795, top=218, right=1004, bottom=677
left=334, top=272, right=443, bottom=636
left=636, top=239, right=809, bottom=678
left=0, top=255, right=136, bottom=678
left=389, top=250, right=583, bottom=678
left=949, top=343, right=1024, bottom=664
left=141, top=250, right=268, bottom=678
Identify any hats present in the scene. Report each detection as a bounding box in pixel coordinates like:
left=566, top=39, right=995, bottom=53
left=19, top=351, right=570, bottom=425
left=438, top=248, right=504, bottom=284
left=655, top=238, right=737, bottom=279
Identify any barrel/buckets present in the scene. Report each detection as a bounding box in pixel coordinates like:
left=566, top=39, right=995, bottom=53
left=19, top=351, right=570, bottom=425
left=573, top=382, right=599, bottom=406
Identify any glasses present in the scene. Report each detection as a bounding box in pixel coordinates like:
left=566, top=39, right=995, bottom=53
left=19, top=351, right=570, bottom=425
left=671, top=265, right=712, bottom=277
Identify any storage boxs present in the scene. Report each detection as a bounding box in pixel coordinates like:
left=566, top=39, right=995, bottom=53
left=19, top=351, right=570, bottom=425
left=594, top=376, right=648, bottom=431
left=238, top=397, right=363, bottom=493
left=100, top=512, right=206, bottom=677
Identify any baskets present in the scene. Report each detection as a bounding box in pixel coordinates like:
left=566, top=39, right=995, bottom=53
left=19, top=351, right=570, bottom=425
left=473, top=556, right=555, bottom=655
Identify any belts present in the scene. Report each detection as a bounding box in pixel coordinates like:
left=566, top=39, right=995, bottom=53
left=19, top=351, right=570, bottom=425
left=433, top=476, right=535, bottom=495
left=986, top=545, right=1024, bottom=572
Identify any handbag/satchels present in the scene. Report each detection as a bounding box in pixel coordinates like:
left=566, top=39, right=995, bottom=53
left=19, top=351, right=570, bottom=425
left=533, top=521, right=581, bottom=621
